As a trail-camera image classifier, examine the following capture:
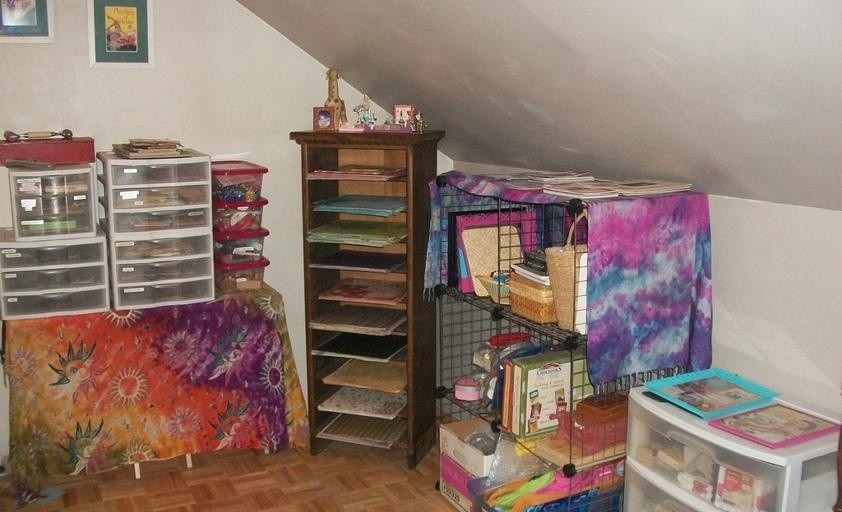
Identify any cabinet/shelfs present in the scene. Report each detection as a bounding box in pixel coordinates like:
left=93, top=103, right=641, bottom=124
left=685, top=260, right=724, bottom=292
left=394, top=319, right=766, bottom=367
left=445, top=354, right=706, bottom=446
left=0, top=146, right=217, bottom=323
left=288, top=128, right=445, bottom=470
left=433, top=174, right=710, bottom=512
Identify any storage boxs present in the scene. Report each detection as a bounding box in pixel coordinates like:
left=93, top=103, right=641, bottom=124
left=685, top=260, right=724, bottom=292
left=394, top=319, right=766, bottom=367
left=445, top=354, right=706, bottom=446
left=211, top=160, right=271, bottom=294
left=621, top=375, right=842, bottom=511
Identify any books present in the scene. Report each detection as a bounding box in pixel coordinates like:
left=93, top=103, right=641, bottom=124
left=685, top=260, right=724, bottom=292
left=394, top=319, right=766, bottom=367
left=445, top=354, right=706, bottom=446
left=658, top=445, right=684, bottom=471
left=112, top=138, right=193, bottom=159
left=510, top=262, right=549, bottom=286
left=482, top=170, right=694, bottom=199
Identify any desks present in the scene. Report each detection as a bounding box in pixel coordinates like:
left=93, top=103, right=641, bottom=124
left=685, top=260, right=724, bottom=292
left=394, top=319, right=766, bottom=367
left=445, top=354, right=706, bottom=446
left=2, top=283, right=284, bottom=480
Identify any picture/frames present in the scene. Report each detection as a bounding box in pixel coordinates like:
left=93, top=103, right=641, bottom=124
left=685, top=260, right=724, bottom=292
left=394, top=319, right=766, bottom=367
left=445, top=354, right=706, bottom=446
left=86, top=0, right=154, bottom=70
left=313, top=106, right=335, bottom=132
left=0, top=0, right=57, bottom=45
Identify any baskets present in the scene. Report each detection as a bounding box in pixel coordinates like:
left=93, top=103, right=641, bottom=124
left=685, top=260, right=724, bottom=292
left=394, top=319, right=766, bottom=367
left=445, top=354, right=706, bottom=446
left=544, top=210, right=592, bottom=336
left=507, top=271, right=558, bottom=325
left=475, top=272, right=511, bottom=306
